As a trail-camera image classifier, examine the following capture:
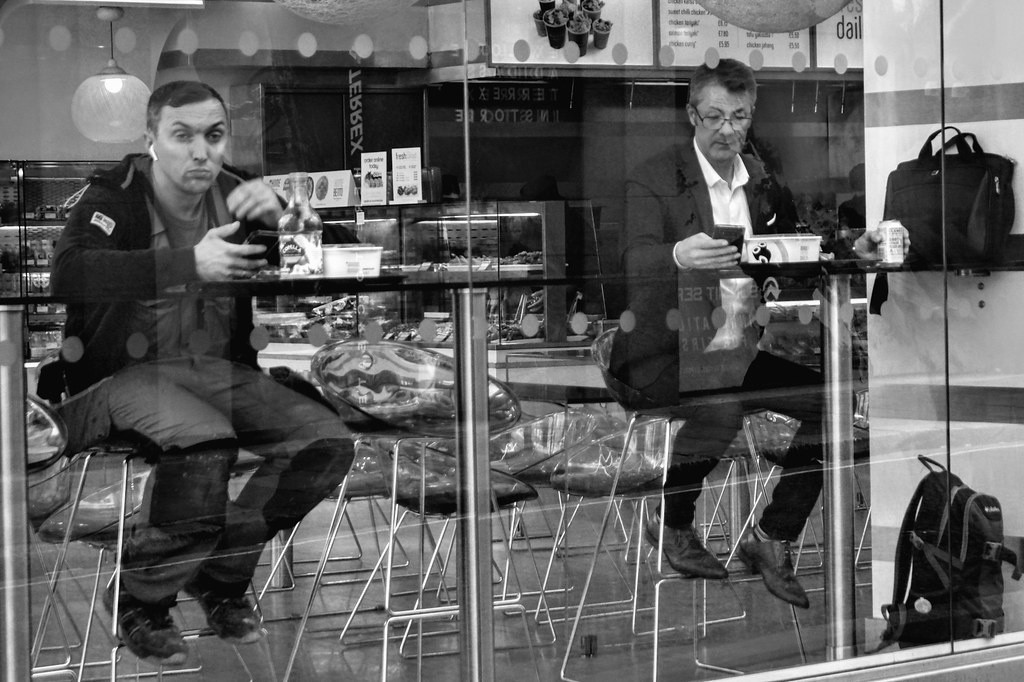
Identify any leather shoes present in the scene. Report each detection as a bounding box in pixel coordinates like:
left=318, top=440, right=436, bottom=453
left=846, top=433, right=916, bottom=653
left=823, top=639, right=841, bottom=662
left=645, top=507, right=729, bottom=580
left=733, top=523, right=810, bottom=610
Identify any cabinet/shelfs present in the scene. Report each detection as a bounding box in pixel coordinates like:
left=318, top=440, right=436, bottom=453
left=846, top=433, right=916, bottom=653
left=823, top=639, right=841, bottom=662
left=0, top=161, right=570, bottom=349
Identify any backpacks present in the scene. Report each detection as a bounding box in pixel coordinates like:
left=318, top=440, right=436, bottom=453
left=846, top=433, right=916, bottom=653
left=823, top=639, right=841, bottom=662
left=865, top=455, right=1023, bottom=654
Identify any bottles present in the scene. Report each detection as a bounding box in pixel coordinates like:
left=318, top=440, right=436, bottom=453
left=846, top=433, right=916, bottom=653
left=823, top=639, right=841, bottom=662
left=280, top=172, right=324, bottom=278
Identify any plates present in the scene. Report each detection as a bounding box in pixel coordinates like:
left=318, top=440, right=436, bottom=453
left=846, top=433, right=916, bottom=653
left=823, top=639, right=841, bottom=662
left=824, top=257, right=883, bottom=267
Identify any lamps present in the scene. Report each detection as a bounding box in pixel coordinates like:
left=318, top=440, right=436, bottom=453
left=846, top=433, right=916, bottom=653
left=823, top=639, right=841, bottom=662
left=71, top=7, right=154, bottom=143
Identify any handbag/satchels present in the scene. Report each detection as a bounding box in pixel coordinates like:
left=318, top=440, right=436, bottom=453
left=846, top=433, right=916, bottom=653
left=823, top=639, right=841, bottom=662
left=878, top=124, right=1016, bottom=268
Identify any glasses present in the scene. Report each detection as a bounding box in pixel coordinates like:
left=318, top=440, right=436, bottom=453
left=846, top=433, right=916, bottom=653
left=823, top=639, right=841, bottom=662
left=690, top=104, right=755, bottom=130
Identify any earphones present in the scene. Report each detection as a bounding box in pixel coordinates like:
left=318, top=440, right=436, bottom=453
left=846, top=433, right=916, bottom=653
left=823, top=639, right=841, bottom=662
left=148, top=144, right=158, bottom=161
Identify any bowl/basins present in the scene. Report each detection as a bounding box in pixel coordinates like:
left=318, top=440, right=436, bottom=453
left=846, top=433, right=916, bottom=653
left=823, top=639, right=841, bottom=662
left=744, top=236, right=823, bottom=263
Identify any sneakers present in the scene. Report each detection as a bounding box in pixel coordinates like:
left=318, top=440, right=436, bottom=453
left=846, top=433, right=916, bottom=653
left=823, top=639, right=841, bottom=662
left=183, top=572, right=266, bottom=643
left=102, top=573, right=189, bottom=670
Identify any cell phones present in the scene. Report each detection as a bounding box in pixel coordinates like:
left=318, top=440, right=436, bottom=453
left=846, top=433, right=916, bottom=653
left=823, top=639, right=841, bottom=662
left=711, top=224, right=745, bottom=266
left=240, top=230, right=280, bottom=259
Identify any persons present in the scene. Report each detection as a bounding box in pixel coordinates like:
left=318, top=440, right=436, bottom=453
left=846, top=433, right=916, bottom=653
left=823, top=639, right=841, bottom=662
left=49, top=80, right=361, bottom=666
left=608, top=58, right=911, bottom=609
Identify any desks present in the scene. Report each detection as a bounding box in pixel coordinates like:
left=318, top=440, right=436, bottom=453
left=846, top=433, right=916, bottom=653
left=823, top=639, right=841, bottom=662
left=0, top=265, right=989, bottom=682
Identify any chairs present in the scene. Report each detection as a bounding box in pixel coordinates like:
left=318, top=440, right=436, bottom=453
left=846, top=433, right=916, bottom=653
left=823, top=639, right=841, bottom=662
left=26, top=323, right=868, bottom=682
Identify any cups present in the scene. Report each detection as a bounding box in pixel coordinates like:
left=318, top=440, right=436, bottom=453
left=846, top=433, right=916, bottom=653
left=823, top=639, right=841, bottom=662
left=533, top=0, right=612, bottom=56
